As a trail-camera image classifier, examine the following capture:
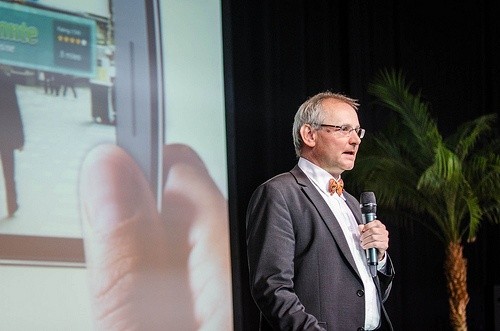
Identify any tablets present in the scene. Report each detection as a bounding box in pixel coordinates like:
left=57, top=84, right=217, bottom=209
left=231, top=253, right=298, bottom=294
left=0, top=0, right=164, bottom=269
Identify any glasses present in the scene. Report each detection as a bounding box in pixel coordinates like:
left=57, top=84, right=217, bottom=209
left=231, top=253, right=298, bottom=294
left=303, top=122, right=365, bottom=139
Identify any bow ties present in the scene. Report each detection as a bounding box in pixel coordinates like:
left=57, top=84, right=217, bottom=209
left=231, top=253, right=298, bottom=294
left=328, top=177, right=344, bottom=196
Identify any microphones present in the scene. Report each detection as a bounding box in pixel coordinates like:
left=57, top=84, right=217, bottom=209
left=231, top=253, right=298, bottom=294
left=359, top=192, right=378, bottom=278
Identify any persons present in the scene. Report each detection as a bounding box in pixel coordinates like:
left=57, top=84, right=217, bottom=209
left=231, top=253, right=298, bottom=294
left=245, top=91, right=397, bottom=331
left=77, top=143, right=233, bottom=330
left=42, top=57, right=107, bottom=98
left=1, top=63, right=28, bottom=219
